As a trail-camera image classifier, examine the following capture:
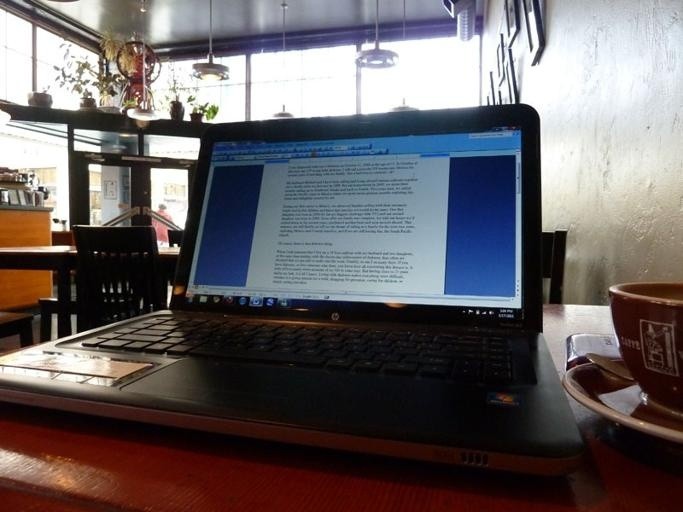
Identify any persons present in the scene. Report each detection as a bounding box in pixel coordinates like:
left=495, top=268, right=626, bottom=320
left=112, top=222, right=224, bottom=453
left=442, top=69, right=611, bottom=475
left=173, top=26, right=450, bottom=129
left=152, top=204, right=173, bottom=248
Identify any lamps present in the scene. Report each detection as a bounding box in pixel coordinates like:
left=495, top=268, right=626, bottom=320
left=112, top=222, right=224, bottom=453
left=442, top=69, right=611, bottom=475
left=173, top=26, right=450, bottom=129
left=269, top=5, right=295, bottom=118
left=189, top=1, right=228, bottom=81
left=353, top=0, right=398, bottom=69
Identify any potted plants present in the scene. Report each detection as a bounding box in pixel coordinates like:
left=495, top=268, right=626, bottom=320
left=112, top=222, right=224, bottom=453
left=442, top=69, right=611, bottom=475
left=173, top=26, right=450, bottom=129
left=161, top=75, right=186, bottom=120
left=54, top=38, right=95, bottom=107
left=185, top=94, right=218, bottom=122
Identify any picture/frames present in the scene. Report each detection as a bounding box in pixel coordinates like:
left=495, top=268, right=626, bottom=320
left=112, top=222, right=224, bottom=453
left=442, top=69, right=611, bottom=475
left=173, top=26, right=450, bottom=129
left=482, top=0, right=546, bottom=104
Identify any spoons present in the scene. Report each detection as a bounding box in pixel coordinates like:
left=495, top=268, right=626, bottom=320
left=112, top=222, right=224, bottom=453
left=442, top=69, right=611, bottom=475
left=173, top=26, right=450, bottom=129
left=585, top=351, right=637, bottom=385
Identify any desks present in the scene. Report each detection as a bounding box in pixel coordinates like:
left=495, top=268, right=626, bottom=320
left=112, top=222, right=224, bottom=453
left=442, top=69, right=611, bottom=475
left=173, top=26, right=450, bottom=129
left=0, top=304, right=683, bottom=512
left=0, top=245, right=180, bottom=339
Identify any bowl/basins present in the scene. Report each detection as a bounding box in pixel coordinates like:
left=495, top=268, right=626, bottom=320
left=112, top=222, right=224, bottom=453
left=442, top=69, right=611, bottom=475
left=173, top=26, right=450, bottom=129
left=607, top=282, right=682, bottom=419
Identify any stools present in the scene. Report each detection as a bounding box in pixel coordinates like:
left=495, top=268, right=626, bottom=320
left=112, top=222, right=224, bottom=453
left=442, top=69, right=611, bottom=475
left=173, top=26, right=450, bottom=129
left=0, top=312, right=34, bottom=351
left=39, top=295, right=129, bottom=343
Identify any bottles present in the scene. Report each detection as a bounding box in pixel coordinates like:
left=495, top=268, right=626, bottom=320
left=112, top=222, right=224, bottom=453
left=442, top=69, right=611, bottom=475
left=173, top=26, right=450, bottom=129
left=0, top=188, right=8, bottom=205
left=37, top=186, right=44, bottom=191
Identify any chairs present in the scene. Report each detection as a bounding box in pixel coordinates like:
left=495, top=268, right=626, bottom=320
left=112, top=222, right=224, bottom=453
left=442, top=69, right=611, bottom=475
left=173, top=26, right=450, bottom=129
left=167, top=228, right=183, bottom=250
left=71, top=221, right=165, bottom=332
left=536, top=226, right=572, bottom=301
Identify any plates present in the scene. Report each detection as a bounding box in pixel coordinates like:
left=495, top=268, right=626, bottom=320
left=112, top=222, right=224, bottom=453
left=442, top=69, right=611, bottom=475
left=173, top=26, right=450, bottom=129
left=562, top=331, right=682, bottom=444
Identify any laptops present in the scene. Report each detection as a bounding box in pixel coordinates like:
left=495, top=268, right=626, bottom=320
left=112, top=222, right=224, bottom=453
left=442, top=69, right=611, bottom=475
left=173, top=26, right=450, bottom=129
left=0, top=104, right=586, bottom=482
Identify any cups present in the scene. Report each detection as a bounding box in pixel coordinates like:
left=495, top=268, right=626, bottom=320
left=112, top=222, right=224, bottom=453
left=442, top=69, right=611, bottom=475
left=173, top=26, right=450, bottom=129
left=15, top=174, right=22, bottom=182
left=24, top=188, right=35, bottom=207
left=35, top=191, right=44, bottom=207
